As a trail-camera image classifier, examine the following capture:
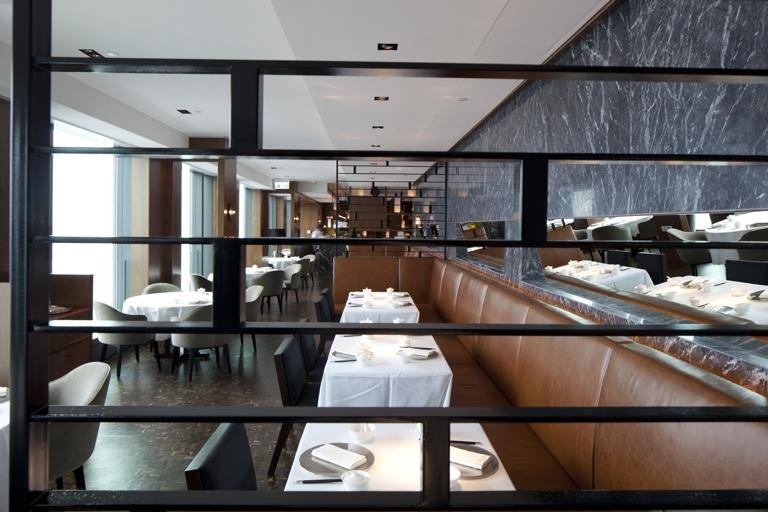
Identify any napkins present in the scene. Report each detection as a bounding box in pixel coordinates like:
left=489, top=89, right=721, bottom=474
left=351, top=292, right=364, bottom=298
left=337, top=345, right=374, bottom=361
left=349, top=300, right=362, bottom=307
left=404, top=347, right=436, bottom=359
left=316, top=444, right=365, bottom=469
left=393, top=291, right=408, bottom=297
left=393, top=299, right=409, bottom=305
left=450, top=445, right=490, bottom=470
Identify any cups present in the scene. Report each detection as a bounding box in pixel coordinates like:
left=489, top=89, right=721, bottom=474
left=634, top=284, right=647, bottom=294
left=544, top=265, right=553, bottom=272
left=450, top=463, right=461, bottom=488
left=702, top=281, right=714, bottom=294
left=197, top=288, right=206, bottom=295
left=386, top=287, right=394, bottom=294
left=358, top=351, right=373, bottom=366
left=362, top=287, right=372, bottom=296
left=340, top=469, right=371, bottom=491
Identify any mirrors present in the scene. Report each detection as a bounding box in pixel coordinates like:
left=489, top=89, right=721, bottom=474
left=455, top=220, right=506, bottom=277
left=416, top=224, right=440, bottom=240
left=521, top=207, right=768, bottom=360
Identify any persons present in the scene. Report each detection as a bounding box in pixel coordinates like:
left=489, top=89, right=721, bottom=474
left=308, top=223, right=330, bottom=238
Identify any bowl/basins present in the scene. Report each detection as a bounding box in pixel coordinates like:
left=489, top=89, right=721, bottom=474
left=347, top=423, right=377, bottom=444
left=734, top=302, right=751, bottom=314
left=689, top=296, right=700, bottom=307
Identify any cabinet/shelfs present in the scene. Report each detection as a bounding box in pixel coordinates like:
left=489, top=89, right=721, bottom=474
left=49, top=273, right=95, bottom=383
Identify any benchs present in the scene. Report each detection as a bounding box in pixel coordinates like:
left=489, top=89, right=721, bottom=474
left=334, top=246, right=768, bottom=490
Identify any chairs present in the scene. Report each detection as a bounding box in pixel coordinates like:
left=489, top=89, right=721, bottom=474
left=187, top=254, right=319, bottom=318
left=267, top=330, right=320, bottom=477
left=168, top=303, right=232, bottom=381
left=313, top=295, right=337, bottom=351
left=183, top=421, right=264, bottom=492
left=238, top=282, right=265, bottom=349
left=321, top=287, right=343, bottom=322
left=92, top=299, right=163, bottom=381
left=142, top=280, right=180, bottom=294
left=48, top=359, right=113, bottom=490
left=295, top=318, right=329, bottom=380
